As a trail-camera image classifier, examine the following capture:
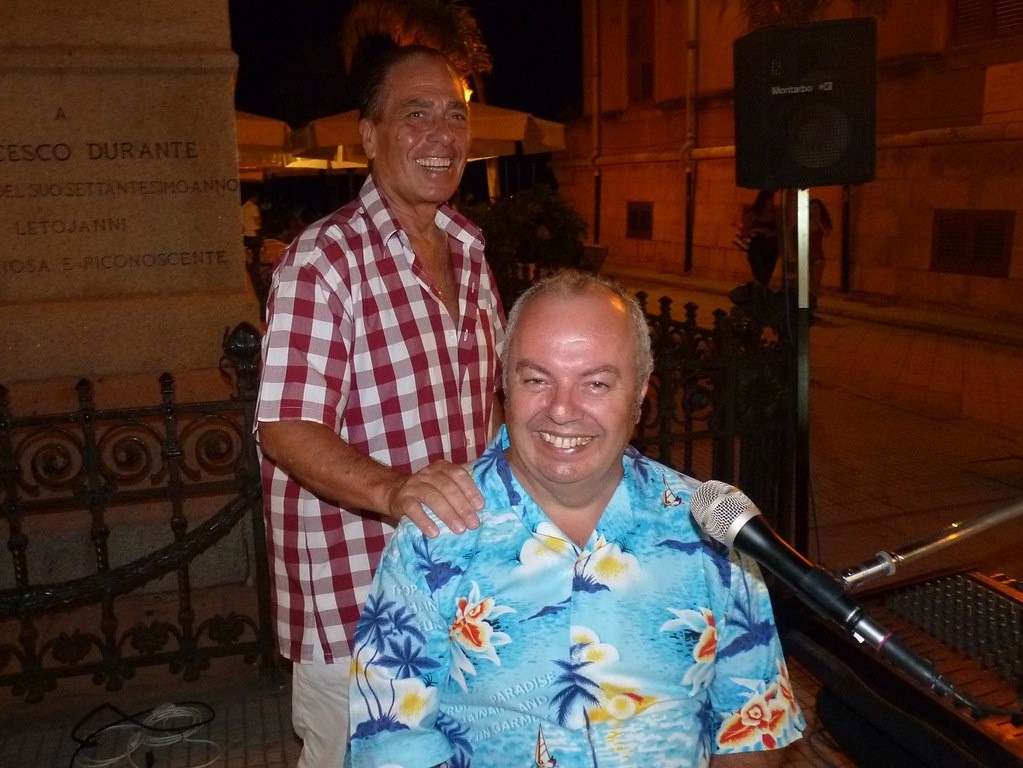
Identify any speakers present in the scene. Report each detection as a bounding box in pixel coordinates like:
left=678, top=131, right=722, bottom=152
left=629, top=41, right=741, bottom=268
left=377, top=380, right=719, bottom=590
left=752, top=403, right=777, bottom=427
left=731, top=16, right=877, bottom=193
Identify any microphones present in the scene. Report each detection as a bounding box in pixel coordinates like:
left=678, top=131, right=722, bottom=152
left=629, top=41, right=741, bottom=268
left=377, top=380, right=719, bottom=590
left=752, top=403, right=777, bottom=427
left=692, top=479, right=956, bottom=698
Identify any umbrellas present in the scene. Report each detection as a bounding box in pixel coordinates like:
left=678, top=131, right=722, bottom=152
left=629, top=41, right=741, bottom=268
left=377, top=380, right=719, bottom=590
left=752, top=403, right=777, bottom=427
left=291, top=96, right=564, bottom=165
left=232, top=110, right=315, bottom=181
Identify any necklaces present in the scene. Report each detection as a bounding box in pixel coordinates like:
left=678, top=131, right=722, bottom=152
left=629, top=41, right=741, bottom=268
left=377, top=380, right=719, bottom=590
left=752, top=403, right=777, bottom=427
left=414, top=232, right=449, bottom=295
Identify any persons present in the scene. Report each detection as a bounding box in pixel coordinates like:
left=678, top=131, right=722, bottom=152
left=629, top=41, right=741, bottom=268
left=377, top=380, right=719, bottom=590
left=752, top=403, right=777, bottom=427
left=350, top=271, right=809, bottom=768
left=241, top=189, right=261, bottom=256
left=742, top=190, right=781, bottom=282
left=809, top=199, right=832, bottom=323
left=249, top=39, right=507, bottom=768
